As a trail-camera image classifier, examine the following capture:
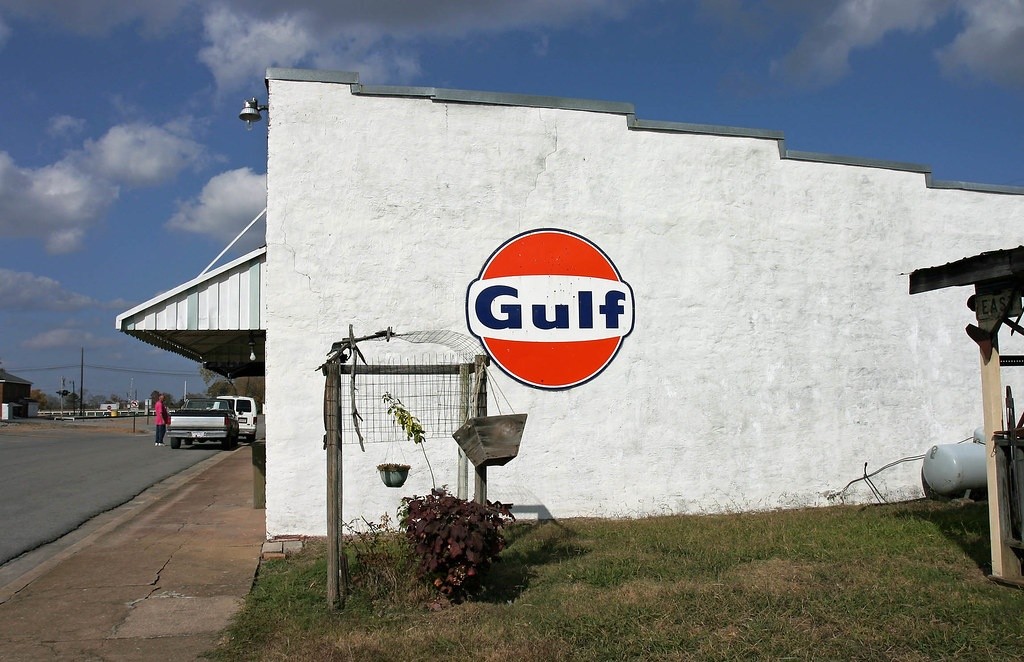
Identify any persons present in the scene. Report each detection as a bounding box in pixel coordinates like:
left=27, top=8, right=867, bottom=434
left=155, top=395, right=170, bottom=447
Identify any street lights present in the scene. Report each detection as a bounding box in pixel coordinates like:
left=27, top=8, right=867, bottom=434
left=68, top=380, right=76, bottom=415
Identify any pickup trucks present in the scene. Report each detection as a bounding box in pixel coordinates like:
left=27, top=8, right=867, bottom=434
left=166, top=398, right=243, bottom=450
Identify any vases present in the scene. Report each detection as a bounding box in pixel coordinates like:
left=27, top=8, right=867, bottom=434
left=453, top=413, right=528, bottom=467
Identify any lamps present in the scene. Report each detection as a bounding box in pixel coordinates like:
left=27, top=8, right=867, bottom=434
left=240, top=97, right=268, bottom=130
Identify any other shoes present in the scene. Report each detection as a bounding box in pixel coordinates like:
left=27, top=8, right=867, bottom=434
left=155, top=443, right=166, bottom=446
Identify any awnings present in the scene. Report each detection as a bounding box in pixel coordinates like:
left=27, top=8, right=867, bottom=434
left=115, top=244, right=266, bottom=379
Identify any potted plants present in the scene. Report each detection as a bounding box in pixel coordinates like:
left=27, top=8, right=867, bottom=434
left=376, top=463, right=412, bottom=488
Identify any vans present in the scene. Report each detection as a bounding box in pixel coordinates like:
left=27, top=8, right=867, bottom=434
left=211, top=395, right=259, bottom=443
